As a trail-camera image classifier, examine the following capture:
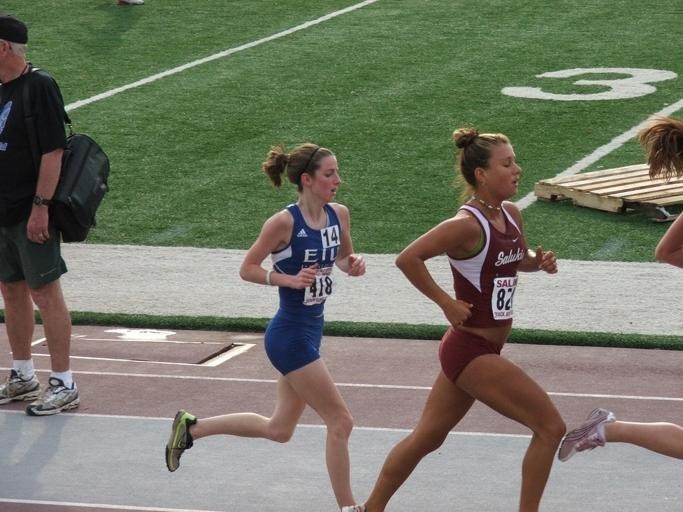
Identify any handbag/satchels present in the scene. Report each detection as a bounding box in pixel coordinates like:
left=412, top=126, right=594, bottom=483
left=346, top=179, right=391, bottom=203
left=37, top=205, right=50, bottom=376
left=22, top=62, right=111, bottom=244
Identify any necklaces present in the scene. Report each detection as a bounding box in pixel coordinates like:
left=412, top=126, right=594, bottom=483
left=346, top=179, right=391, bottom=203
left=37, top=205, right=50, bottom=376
left=471, top=195, right=503, bottom=213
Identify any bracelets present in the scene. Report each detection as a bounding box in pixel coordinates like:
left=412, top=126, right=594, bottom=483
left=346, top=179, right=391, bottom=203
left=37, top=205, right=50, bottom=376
left=265, top=269, right=275, bottom=285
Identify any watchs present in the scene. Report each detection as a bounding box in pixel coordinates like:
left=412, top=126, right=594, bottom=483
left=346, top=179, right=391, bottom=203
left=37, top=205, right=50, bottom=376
left=33, top=195, right=53, bottom=208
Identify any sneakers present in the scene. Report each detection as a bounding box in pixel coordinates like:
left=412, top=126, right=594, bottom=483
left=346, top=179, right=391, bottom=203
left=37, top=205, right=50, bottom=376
left=25, top=377, right=80, bottom=416
left=556, top=407, right=616, bottom=461
left=164, top=407, right=197, bottom=472
left=0, top=368, right=40, bottom=405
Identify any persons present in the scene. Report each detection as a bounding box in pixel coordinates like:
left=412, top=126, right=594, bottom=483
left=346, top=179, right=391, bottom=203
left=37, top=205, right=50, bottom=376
left=0, top=15, right=82, bottom=417
left=557, top=116, right=683, bottom=464
left=339, top=126, right=567, bottom=512
left=165, top=142, right=358, bottom=512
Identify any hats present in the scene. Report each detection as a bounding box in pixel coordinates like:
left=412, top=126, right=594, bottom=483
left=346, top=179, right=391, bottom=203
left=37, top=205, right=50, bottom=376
left=0, top=16, right=27, bottom=45
